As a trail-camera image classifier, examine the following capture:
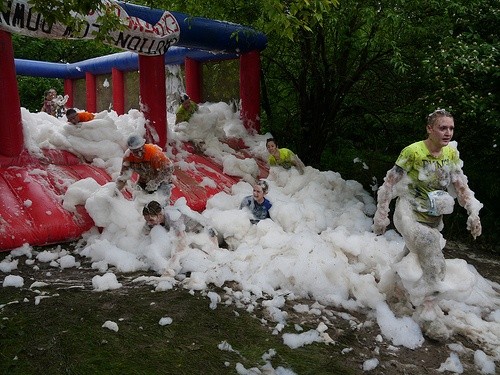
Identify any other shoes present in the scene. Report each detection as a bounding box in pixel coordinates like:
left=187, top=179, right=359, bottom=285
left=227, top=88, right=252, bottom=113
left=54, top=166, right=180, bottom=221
left=418, top=319, right=449, bottom=340
left=387, top=288, right=413, bottom=317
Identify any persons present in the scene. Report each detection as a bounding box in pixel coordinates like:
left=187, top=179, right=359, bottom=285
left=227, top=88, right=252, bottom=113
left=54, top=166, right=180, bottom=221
left=43, top=91, right=60, bottom=117
left=265, top=138, right=305, bottom=175
left=175, top=92, right=199, bottom=126
left=115, top=133, right=173, bottom=208
left=141, top=202, right=229, bottom=277
left=47, top=88, right=69, bottom=117
left=238, top=180, right=273, bottom=225
left=371, top=107, right=482, bottom=341
left=65, top=109, right=96, bottom=125
